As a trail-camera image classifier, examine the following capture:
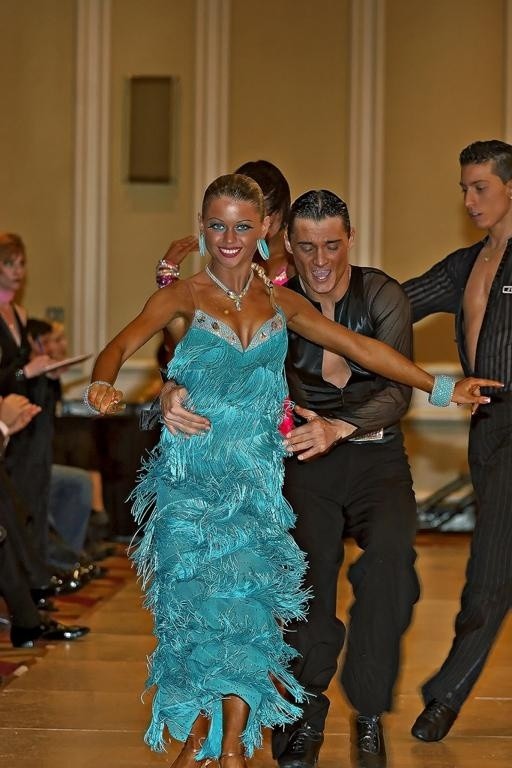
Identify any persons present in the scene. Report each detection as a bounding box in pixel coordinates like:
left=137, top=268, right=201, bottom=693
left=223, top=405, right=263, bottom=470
left=159, top=190, right=415, bottom=767
left=0, top=234, right=83, bottom=609
left=401, top=140, right=511, bottom=741
left=0, top=393, right=90, bottom=647
left=43, top=320, right=120, bottom=562
left=27, top=322, right=107, bottom=576
left=157, top=160, right=298, bottom=293
left=85, top=176, right=504, bottom=767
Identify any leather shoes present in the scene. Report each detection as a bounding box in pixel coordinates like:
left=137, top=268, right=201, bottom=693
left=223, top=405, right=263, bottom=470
left=413, top=700, right=458, bottom=741
left=349, top=714, right=387, bottom=767
left=278, top=724, right=324, bottom=767
left=11, top=617, right=91, bottom=646
left=27, top=559, right=111, bottom=610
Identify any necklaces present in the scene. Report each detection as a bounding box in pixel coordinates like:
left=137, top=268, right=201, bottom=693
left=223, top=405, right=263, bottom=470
left=205, top=263, right=255, bottom=312
left=484, top=235, right=507, bottom=262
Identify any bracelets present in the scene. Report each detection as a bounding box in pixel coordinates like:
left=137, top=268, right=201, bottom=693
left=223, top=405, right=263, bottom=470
left=85, top=379, right=112, bottom=415
left=155, top=259, right=179, bottom=290
left=429, top=372, right=456, bottom=410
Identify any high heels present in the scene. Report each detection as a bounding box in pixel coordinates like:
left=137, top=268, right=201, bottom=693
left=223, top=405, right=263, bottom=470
left=218, top=751, right=249, bottom=768
left=166, top=733, right=213, bottom=768
